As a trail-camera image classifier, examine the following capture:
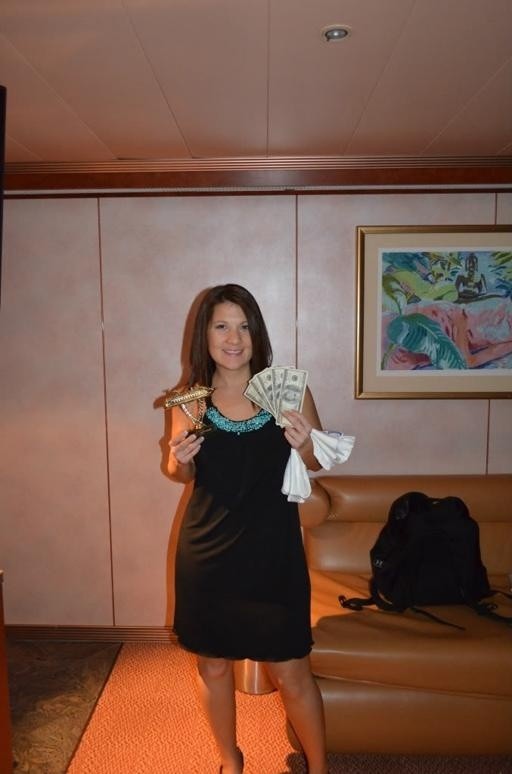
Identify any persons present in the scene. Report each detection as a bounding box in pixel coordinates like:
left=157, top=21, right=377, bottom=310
left=167, top=283, right=328, bottom=774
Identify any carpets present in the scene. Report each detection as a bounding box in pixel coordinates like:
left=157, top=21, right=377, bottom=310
left=5, top=641, right=123, bottom=774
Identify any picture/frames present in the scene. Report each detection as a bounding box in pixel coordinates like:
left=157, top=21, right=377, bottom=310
left=354, top=222, right=511, bottom=398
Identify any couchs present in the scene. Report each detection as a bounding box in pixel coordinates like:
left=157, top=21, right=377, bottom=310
left=286, top=473, right=512, bottom=756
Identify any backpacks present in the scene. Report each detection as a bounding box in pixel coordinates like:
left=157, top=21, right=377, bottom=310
left=367, top=491, right=492, bottom=615
